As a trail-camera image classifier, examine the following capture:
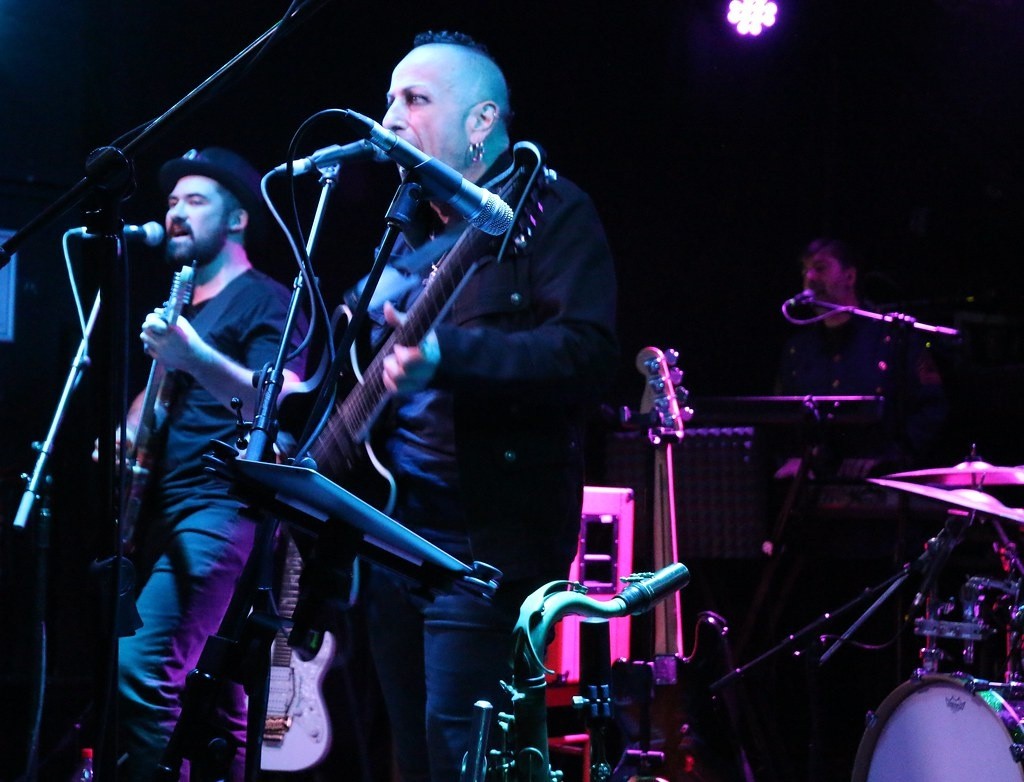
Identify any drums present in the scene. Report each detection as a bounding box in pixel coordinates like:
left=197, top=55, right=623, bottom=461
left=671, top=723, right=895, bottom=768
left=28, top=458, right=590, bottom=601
left=849, top=670, right=1023, bottom=782
left=903, top=580, right=1024, bottom=677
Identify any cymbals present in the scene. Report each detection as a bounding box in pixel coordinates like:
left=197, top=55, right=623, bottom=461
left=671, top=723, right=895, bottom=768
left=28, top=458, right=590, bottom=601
left=868, top=475, right=1023, bottom=525
left=883, top=461, right=1024, bottom=491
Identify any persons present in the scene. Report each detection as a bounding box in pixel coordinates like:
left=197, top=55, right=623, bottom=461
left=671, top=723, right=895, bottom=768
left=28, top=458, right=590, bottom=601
left=94, top=30, right=620, bottom=782
left=772, top=239, right=946, bottom=782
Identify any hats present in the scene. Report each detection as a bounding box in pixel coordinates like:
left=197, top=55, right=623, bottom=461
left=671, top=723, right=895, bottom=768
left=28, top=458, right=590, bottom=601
left=158, top=149, right=272, bottom=238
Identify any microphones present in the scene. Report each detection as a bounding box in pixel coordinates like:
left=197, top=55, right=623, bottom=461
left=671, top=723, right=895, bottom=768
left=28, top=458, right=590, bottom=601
left=792, top=288, right=817, bottom=307
left=80, top=221, right=166, bottom=247
left=340, top=107, right=514, bottom=236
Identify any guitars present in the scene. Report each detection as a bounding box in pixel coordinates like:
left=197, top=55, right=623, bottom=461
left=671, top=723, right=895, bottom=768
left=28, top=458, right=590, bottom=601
left=223, top=538, right=341, bottom=774
left=104, top=257, right=196, bottom=599
left=608, top=342, right=749, bottom=782
left=260, top=138, right=550, bottom=614
